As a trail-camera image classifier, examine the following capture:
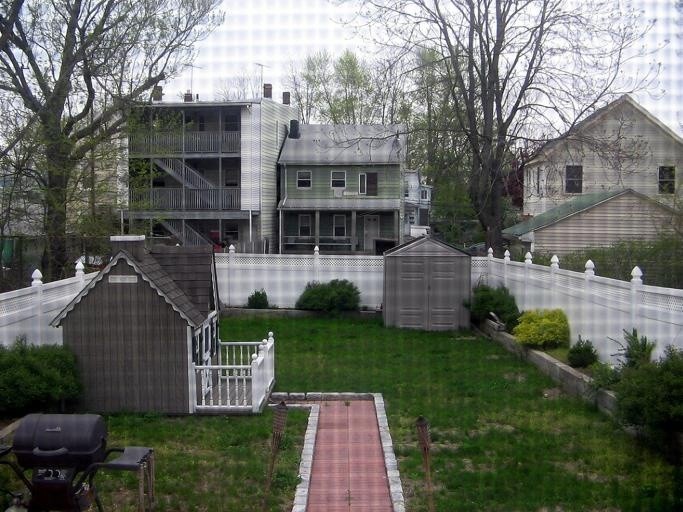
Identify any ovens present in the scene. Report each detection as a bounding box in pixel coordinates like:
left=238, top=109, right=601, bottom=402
left=12, top=412, right=107, bottom=496
